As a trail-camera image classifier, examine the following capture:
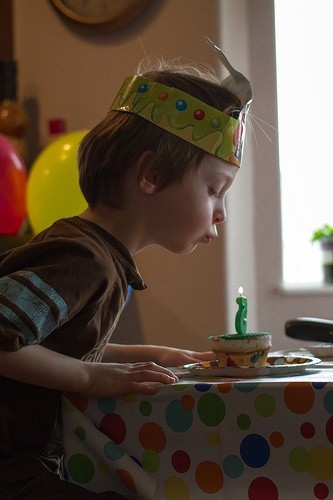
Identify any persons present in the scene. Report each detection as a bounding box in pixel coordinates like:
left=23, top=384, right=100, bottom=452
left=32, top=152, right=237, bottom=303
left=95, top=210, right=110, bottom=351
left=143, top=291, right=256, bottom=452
left=0, top=70, right=246, bottom=500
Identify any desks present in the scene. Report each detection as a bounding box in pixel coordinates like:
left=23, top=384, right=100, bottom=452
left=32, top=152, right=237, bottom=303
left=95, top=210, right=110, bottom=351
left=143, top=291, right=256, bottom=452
left=61, top=356, right=333, bottom=500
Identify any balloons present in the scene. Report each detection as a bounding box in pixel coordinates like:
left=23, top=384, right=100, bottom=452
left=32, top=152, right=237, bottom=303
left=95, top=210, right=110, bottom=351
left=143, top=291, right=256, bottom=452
left=27, top=121, right=98, bottom=234
left=0, top=132, right=26, bottom=234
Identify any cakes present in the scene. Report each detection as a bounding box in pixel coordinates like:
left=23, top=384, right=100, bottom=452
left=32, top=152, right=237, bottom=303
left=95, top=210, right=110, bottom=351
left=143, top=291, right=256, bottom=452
left=208, top=332, right=273, bottom=367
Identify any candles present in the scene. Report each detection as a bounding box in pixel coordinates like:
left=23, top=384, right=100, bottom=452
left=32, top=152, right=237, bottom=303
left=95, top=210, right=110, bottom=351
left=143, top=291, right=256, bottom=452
left=234, top=287, right=249, bottom=334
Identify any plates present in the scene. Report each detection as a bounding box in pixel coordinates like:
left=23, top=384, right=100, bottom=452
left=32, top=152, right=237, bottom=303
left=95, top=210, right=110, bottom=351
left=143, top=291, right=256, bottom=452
left=184, top=356, right=321, bottom=376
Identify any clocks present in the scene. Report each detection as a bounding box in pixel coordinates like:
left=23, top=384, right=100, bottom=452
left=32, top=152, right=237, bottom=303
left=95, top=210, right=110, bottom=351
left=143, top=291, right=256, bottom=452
left=50, top=0, right=152, bottom=36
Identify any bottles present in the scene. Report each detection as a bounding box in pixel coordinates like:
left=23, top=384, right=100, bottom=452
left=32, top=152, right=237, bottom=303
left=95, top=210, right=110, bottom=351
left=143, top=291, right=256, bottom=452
left=44, top=118, right=67, bottom=145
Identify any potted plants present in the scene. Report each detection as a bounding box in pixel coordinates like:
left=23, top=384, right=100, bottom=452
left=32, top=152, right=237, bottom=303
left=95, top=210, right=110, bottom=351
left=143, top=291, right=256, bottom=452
left=312, top=224, right=333, bottom=284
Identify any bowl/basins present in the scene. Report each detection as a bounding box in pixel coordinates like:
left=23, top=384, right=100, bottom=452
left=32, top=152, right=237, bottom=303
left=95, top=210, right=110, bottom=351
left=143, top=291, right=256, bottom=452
left=303, top=345, right=333, bottom=357
